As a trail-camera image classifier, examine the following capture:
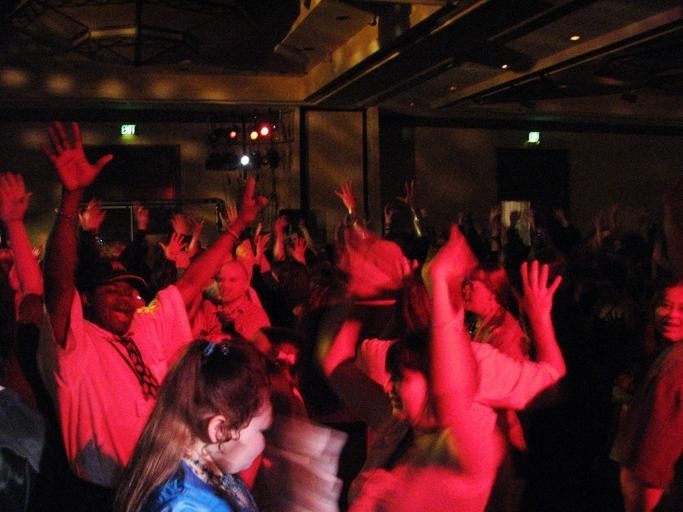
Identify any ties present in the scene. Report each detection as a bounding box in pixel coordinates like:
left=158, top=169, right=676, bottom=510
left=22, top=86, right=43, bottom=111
left=122, top=336, right=161, bottom=398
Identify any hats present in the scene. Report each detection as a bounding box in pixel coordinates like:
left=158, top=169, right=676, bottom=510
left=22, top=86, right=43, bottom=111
left=77, top=255, right=150, bottom=290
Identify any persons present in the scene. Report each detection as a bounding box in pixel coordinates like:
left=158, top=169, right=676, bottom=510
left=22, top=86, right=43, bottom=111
left=2, top=117, right=682, bottom=510
